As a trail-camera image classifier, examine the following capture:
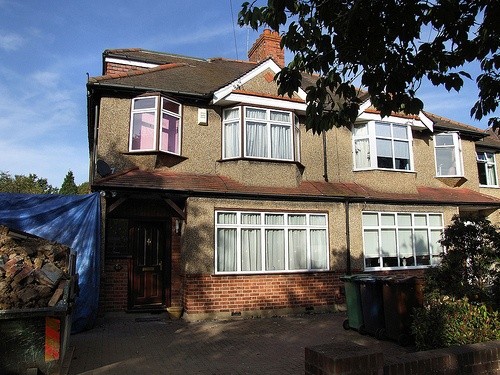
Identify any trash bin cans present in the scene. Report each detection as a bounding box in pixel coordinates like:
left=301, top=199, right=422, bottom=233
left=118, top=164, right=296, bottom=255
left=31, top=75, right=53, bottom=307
left=0, top=304, right=68, bottom=375
left=339, top=273, right=372, bottom=334
left=380, top=274, right=420, bottom=344
left=355, top=276, right=388, bottom=340
